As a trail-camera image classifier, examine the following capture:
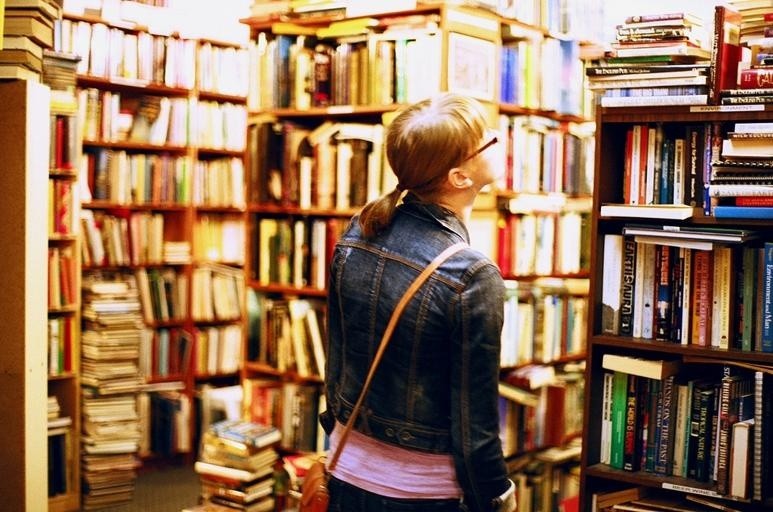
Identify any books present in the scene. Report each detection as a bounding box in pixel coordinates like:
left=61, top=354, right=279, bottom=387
left=0, top=0, right=772, bottom=511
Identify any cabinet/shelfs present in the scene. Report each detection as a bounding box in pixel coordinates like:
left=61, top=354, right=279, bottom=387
left=47, top=113, right=81, bottom=507
left=245, top=16, right=438, bottom=450
left=64, top=13, right=242, bottom=388
left=500, top=23, right=591, bottom=512
left=579, top=107, right=767, bottom=510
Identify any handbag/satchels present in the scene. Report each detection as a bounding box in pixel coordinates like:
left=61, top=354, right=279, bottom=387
left=297, top=462, right=330, bottom=512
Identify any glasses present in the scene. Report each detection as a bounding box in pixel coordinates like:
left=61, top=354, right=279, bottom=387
left=455, top=133, right=501, bottom=168
